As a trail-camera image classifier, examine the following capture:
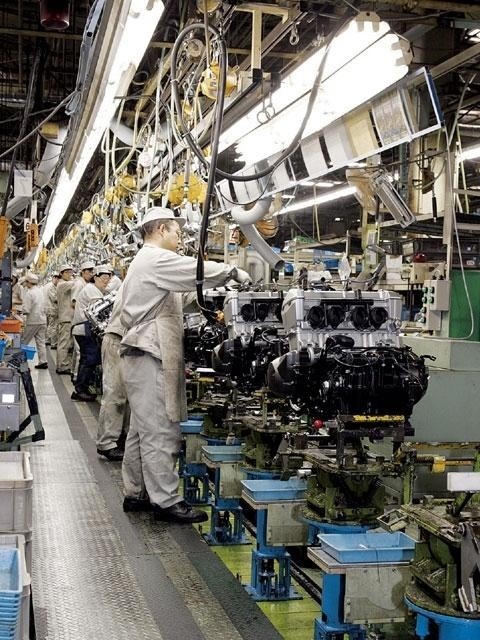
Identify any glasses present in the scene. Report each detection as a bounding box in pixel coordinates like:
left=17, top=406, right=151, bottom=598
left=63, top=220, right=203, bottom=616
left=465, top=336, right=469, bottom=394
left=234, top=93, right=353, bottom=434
left=98, top=276, right=111, bottom=282
left=62, top=270, right=74, bottom=276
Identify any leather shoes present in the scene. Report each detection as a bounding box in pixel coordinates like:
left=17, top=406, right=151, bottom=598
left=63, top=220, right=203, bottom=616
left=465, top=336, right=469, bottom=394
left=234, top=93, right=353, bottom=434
left=35, top=363, right=48, bottom=369
left=51, top=346, right=58, bottom=350
left=117, top=439, right=125, bottom=450
left=124, top=498, right=154, bottom=512
left=56, top=369, right=59, bottom=373
left=75, top=390, right=97, bottom=401
left=71, top=373, right=74, bottom=381
left=58, top=370, right=72, bottom=375
left=97, top=447, right=124, bottom=459
left=71, top=392, right=79, bottom=400
left=45, top=342, right=52, bottom=346
left=73, top=380, right=77, bottom=385
left=155, top=499, right=209, bottom=523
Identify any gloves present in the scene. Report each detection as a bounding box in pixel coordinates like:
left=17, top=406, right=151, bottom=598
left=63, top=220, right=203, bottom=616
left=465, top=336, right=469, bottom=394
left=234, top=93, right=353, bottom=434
left=235, top=268, right=253, bottom=286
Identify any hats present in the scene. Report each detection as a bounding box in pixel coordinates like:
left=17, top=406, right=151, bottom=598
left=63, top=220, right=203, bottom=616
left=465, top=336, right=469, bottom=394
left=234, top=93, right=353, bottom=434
left=91, top=264, right=113, bottom=276
left=139, top=206, right=186, bottom=228
left=107, top=264, right=114, bottom=274
left=60, top=265, right=74, bottom=273
left=12, top=272, right=20, bottom=279
left=53, top=271, right=61, bottom=277
left=79, top=262, right=98, bottom=274
left=22, top=276, right=28, bottom=283
left=26, top=273, right=39, bottom=283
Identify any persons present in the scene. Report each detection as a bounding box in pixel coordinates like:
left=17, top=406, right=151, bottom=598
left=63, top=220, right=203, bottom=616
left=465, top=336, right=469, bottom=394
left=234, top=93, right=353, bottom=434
left=41, top=272, right=61, bottom=350
left=55, top=264, right=77, bottom=375
left=118, top=206, right=252, bottom=524
left=16, top=273, right=50, bottom=369
left=102, top=265, right=122, bottom=297
left=8, top=276, right=26, bottom=321
left=69, top=265, right=113, bottom=403
left=95, top=275, right=129, bottom=462
left=68, top=262, right=97, bottom=385
